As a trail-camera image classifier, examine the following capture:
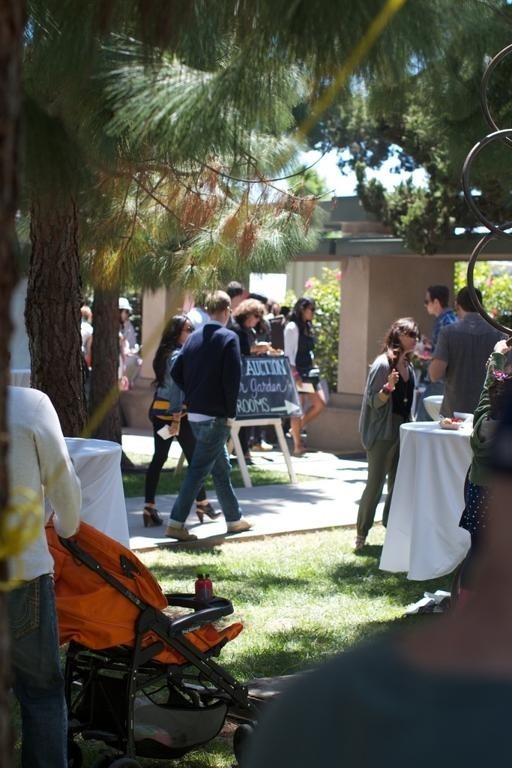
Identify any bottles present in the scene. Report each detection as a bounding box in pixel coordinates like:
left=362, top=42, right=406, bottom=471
left=194, top=566, right=212, bottom=599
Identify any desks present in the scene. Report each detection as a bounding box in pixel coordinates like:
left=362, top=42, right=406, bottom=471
left=377, top=420, right=470, bottom=581
left=46, top=437, right=128, bottom=546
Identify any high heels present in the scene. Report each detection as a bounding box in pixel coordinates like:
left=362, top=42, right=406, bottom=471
left=196, top=503, right=222, bottom=524
left=143, top=507, right=163, bottom=528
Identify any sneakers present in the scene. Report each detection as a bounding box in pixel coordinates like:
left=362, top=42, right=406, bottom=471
left=252, top=440, right=274, bottom=451
left=165, top=526, right=197, bottom=540
left=227, top=520, right=252, bottom=533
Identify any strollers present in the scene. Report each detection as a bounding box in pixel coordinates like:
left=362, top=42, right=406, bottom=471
left=37, top=505, right=264, bottom=763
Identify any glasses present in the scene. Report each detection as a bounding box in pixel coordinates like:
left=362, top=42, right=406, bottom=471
left=407, top=331, right=420, bottom=343
left=187, top=325, right=195, bottom=333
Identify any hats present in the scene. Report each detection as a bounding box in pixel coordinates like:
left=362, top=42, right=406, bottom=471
left=119, top=297, right=133, bottom=314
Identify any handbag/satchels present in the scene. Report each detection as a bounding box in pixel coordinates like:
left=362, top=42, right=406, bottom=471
left=296, top=381, right=315, bottom=394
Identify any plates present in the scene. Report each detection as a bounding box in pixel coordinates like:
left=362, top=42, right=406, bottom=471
left=440, top=421, right=462, bottom=430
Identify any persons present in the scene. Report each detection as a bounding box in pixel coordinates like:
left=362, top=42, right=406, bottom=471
left=115, top=296, right=134, bottom=387
left=185, top=280, right=327, bottom=467
left=238, top=406, right=510, bottom=767
left=79, top=305, right=94, bottom=359
left=353, top=317, right=432, bottom=550
left=462, top=341, right=511, bottom=583
left=86, top=324, right=123, bottom=385
left=1, top=385, right=82, bottom=767
left=426, top=286, right=506, bottom=417
left=142, top=312, right=222, bottom=528
left=167, top=289, right=250, bottom=543
left=413, top=285, right=456, bottom=422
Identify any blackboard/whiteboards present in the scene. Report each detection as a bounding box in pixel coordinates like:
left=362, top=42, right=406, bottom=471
left=235, top=354, right=302, bottom=420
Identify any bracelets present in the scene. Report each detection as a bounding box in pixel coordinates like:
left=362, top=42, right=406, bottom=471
left=173, top=416, right=180, bottom=422
left=383, top=387, right=394, bottom=395
left=387, top=382, right=395, bottom=389
